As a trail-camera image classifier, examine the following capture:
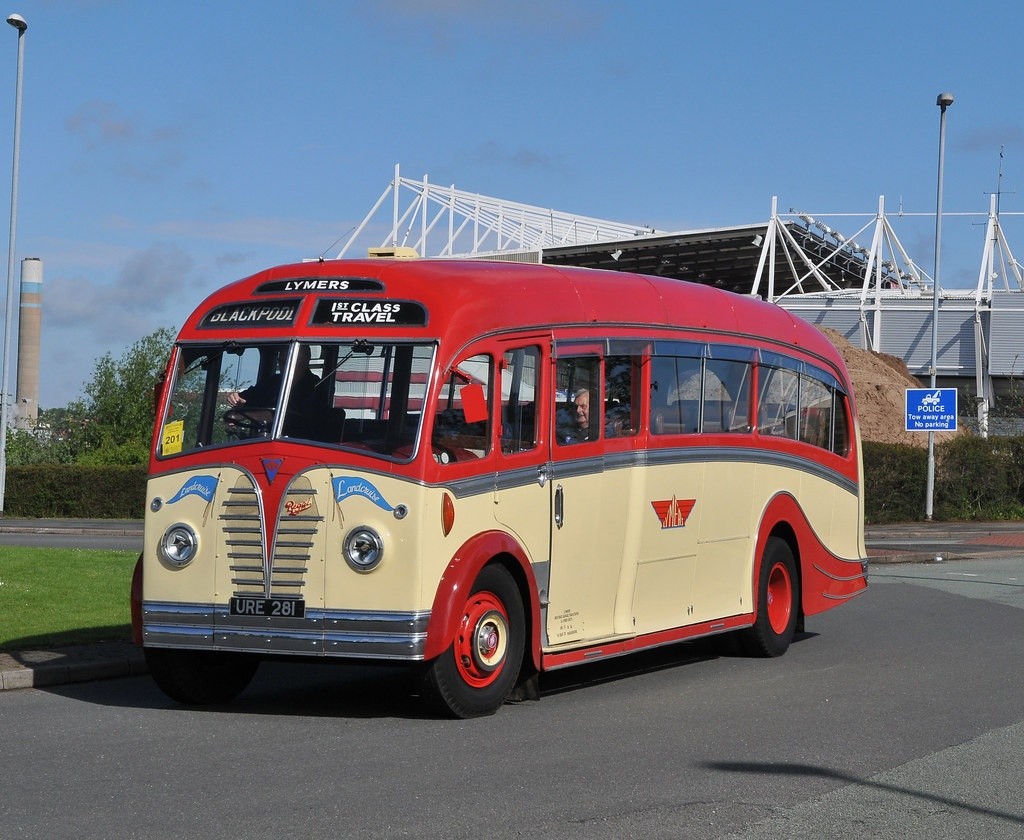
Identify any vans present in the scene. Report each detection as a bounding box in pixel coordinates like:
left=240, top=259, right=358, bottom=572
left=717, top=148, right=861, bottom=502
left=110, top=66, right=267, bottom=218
left=131, top=246, right=869, bottom=717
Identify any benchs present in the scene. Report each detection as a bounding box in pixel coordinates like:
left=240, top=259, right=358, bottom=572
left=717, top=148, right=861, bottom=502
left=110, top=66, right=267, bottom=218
left=768, top=403, right=798, bottom=439
left=346, top=417, right=396, bottom=445
left=503, top=405, right=536, bottom=434
left=442, top=434, right=534, bottom=458
left=531, top=402, right=577, bottom=414
left=442, top=408, right=491, bottom=434
left=649, top=409, right=664, bottom=435
left=801, top=409, right=828, bottom=445
left=672, top=401, right=735, bottom=432
left=606, top=419, right=633, bottom=436
left=401, top=413, right=442, bottom=444
left=735, top=401, right=767, bottom=436
left=660, top=407, right=690, bottom=434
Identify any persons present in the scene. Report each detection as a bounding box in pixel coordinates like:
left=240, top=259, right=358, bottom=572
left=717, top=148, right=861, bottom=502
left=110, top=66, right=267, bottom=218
left=564, top=387, right=610, bottom=445
left=227, top=345, right=329, bottom=431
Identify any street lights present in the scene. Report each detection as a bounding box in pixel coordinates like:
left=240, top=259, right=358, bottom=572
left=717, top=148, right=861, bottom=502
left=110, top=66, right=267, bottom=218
left=0, top=14, right=28, bottom=518
left=905, top=93, right=957, bottom=521
